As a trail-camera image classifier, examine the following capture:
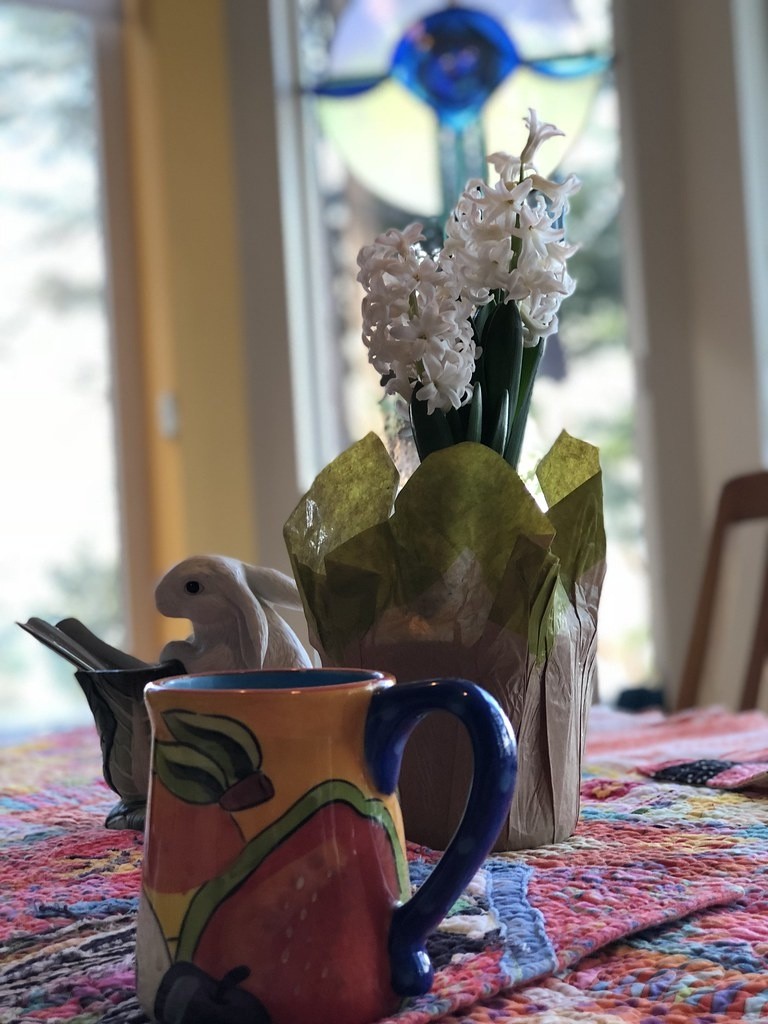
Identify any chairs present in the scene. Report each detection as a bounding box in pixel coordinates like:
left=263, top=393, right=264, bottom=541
left=674, top=471, right=767, bottom=710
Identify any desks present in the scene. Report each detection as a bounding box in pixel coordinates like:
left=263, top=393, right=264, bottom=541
left=0, top=705, right=768, bottom=1024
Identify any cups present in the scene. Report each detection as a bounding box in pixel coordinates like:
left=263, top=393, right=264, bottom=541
left=72, top=664, right=186, bottom=828
left=136, top=667, right=517, bottom=1024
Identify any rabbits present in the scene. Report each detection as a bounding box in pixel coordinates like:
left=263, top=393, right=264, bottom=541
left=154, top=554, right=313, bottom=676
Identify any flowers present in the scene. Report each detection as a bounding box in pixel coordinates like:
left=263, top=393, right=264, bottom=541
left=354, top=110, right=583, bottom=481
